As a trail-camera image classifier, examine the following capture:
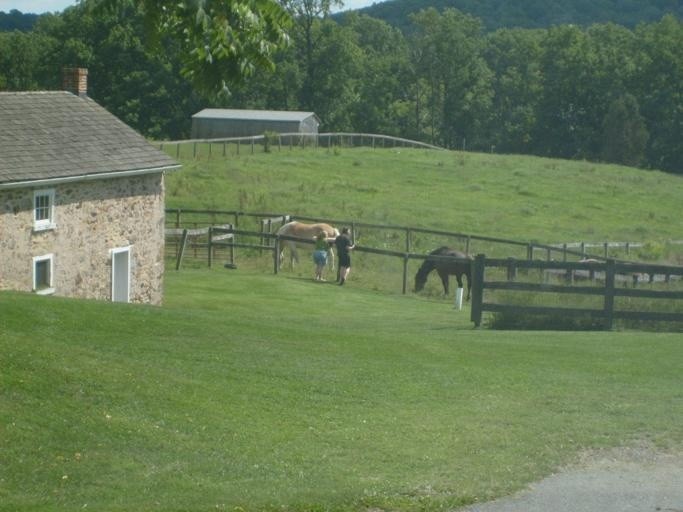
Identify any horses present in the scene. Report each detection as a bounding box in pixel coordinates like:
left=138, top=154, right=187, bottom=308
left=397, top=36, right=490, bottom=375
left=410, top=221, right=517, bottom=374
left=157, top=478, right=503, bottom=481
left=273, top=219, right=342, bottom=271
left=412, top=245, right=474, bottom=303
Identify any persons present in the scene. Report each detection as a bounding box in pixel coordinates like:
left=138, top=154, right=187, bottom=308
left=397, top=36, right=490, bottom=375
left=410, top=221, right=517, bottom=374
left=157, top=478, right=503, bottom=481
left=312, top=231, right=330, bottom=282
left=335, top=227, right=355, bottom=284
